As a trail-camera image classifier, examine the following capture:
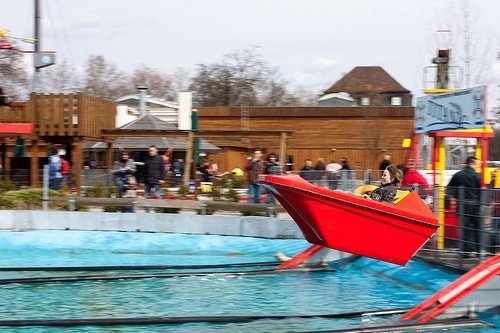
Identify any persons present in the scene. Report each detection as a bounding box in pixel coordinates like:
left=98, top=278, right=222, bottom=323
left=361, top=164, right=403, bottom=203
left=198, top=163, right=215, bottom=182
left=243, top=148, right=265, bottom=203
left=46, top=145, right=70, bottom=191
left=447, top=156, right=496, bottom=258
left=109, top=150, right=136, bottom=213
left=399, top=165, right=429, bottom=194
left=300, top=155, right=392, bottom=190
left=262, top=153, right=283, bottom=218
left=143, top=146, right=166, bottom=213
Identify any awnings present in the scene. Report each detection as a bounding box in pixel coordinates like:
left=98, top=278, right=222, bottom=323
left=0, top=122, right=36, bottom=135
